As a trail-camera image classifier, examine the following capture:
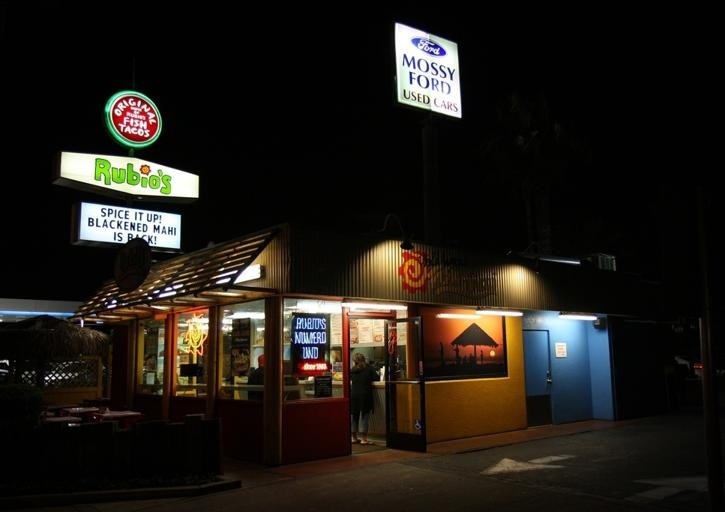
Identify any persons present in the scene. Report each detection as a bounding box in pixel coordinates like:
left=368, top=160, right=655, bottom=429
left=350, top=353, right=380, bottom=445
left=247, top=353, right=265, bottom=399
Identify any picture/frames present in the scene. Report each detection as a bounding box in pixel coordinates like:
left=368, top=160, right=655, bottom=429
left=38, top=407, right=140, bottom=433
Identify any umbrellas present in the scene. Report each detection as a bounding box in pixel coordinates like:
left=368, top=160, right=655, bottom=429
left=351, top=438, right=375, bottom=444
